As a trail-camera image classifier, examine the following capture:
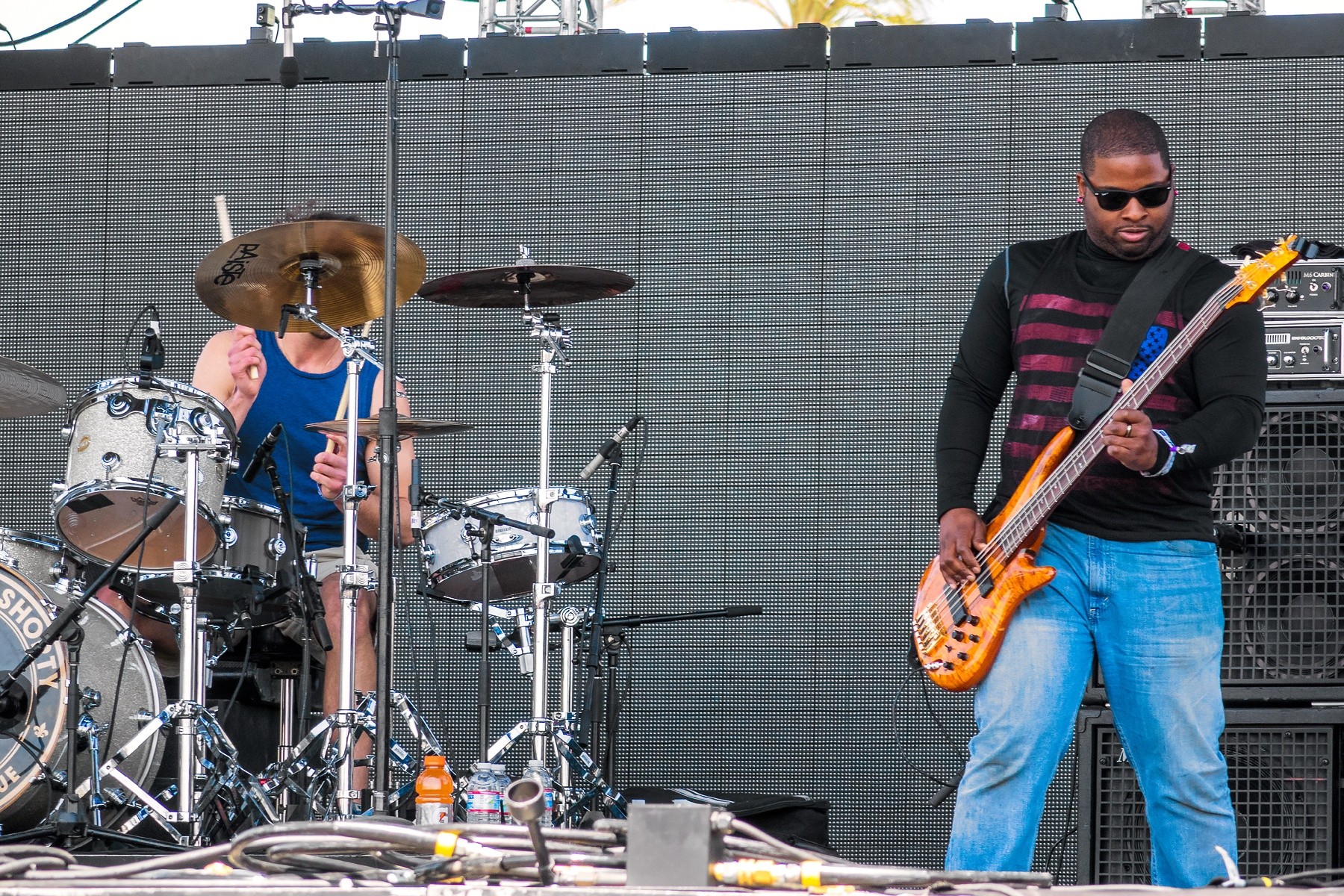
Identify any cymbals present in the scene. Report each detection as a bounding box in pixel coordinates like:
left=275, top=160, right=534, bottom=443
left=0, top=355, right=68, bottom=420
left=195, top=220, right=426, bottom=333
left=303, top=414, right=474, bottom=442
left=418, top=265, right=636, bottom=309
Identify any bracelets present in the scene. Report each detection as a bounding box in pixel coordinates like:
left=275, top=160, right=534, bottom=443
left=316, top=483, right=342, bottom=501
left=1140, top=429, right=1195, bottom=478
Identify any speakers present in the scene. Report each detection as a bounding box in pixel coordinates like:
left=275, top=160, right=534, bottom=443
left=1076, top=707, right=1344, bottom=888
left=1076, top=387, right=1344, bottom=702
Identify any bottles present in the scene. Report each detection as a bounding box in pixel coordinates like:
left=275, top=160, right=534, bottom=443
left=464, top=759, right=553, bottom=830
left=413, top=755, right=455, bottom=826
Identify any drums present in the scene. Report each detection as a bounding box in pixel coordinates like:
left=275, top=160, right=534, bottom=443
left=49, top=376, right=238, bottom=573
left=418, top=485, right=602, bottom=604
left=121, top=494, right=308, bottom=630
left=0, top=562, right=169, bottom=852
left=0, top=527, right=89, bottom=590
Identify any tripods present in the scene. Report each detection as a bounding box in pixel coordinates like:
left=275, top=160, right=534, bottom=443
left=0, top=254, right=632, bottom=856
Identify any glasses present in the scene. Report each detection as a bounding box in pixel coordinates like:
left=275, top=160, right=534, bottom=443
left=1084, top=165, right=1172, bottom=211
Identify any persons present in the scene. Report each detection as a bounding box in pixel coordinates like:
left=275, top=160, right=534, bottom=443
left=936, top=108, right=1267, bottom=886
left=96, top=211, right=420, bottom=816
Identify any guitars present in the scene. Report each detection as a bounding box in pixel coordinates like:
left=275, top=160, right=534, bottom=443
left=911, top=231, right=1303, bottom=692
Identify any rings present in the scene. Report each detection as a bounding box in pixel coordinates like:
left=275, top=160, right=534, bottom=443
left=1123, top=423, right=1132, bottom=437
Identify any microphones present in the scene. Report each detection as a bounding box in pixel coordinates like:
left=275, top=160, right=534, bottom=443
left=411, top=458, right=423, bottom=538
left=145, top=310, right=165, bottom=369
left=280, top=0, right=300, bottom=91
left=243, top=422, right=282, bottom=483
left=0, top=687, right=19, bottom=719
left=464, top=629, right=556, bottom=653
left=579, top=416, right=639, bottom=479
